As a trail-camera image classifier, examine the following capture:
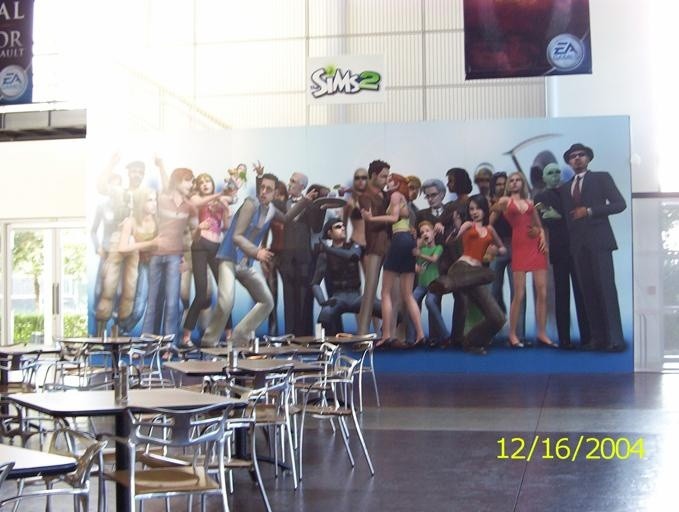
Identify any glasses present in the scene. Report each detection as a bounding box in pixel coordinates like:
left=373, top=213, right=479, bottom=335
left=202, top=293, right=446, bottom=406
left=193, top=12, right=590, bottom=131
left=569, top=152, right=586, bottom=158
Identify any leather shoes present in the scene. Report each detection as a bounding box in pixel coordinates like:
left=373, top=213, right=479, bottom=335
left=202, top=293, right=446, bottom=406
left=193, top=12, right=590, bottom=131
left=506, top=334, right=626, bottom=352
left=375, top=333, right=495, bottom=355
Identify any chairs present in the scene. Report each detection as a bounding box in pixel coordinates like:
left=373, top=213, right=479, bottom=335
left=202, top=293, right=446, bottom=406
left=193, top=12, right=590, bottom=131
left=1, top=330, right=382, bottom=511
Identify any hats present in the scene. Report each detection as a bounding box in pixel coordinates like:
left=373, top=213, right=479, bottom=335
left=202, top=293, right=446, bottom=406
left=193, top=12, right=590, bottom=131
left=564, top=144, right=593, bottom=163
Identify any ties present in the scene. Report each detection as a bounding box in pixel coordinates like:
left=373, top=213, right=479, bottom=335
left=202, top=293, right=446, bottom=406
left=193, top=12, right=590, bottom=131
left=574, top=178, right=581, bottom=204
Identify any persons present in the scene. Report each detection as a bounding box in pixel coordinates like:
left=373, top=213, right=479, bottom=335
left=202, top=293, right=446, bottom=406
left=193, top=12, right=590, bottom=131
left=92, top=144, right=627, bottom=359
